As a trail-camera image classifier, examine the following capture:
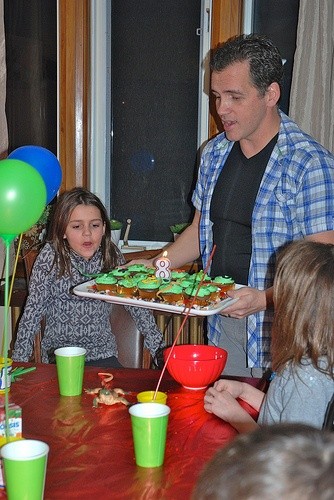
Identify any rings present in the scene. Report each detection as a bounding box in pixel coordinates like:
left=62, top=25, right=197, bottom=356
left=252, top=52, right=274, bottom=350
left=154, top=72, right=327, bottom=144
left=228, top=314, right=230, bottom=317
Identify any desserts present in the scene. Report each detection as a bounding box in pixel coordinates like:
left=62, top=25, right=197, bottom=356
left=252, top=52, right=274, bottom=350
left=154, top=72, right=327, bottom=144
left=93, top=264, right=235, bottom=308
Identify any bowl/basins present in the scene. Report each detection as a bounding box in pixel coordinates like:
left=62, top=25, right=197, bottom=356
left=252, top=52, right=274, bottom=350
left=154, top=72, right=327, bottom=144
left=163, top=344, right=228, bottom=390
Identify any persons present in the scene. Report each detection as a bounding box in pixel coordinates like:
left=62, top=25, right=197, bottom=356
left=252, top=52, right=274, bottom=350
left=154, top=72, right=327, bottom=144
left=204, top=242, right=334, bottom=435
left=194, top=421, right=334, bottom=500
left=116, top=33, right=334, bottom=381
left=11, top=187, right=170, bottom=371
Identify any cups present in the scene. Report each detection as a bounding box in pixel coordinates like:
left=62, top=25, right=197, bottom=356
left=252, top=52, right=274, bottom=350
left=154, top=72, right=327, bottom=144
left=0, top=439, right=50, bottom=500
left=136, top=390, right=167, bottom=405
left=54, top=346, right=85, bottom=396
left=0, top=357, right=13, bottom=393
left=128, top=402, right=170, bottom=468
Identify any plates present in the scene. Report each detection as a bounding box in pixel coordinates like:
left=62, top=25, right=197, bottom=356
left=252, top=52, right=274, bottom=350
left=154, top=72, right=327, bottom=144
left=72, top=278, right=249, bottom=316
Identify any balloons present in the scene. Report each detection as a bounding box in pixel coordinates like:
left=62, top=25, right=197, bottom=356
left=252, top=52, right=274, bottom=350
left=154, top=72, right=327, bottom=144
left=7, top=145, right=63, bottom=204
left=0, top=158, right=48, bottom=244
